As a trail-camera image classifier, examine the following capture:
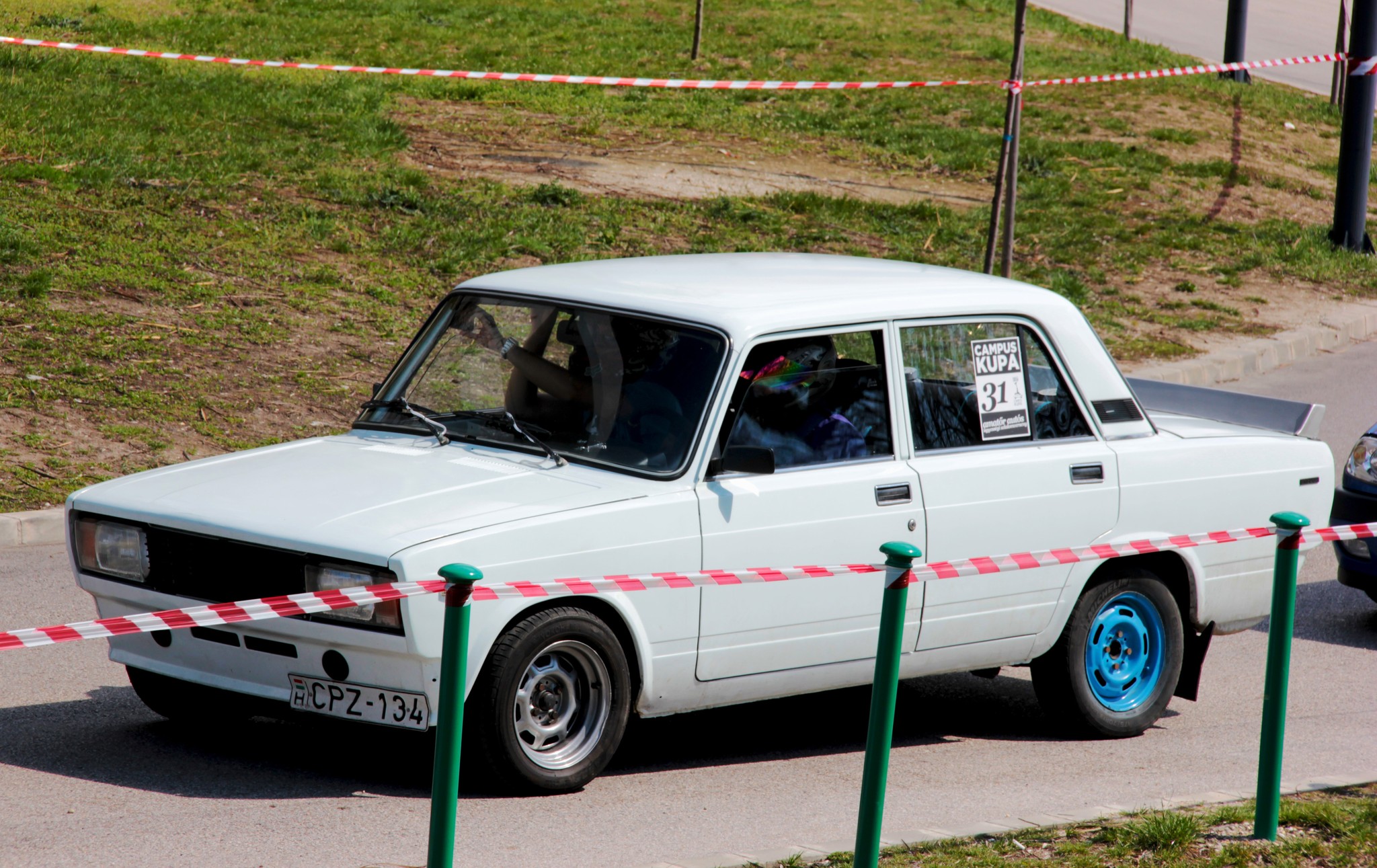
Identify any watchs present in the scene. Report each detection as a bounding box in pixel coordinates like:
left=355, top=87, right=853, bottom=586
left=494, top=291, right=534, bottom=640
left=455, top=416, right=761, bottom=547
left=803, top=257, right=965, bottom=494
left=500, top=336, right=519, bottom=360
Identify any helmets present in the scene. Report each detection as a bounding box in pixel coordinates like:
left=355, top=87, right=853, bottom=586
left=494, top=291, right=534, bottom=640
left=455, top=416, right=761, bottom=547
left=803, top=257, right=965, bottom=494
left=754, top=335, right=837, bottom=408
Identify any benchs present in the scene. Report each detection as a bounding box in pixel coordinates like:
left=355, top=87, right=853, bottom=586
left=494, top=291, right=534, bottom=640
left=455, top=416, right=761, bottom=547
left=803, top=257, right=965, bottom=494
left=907, top=378, right=1084, bottom=450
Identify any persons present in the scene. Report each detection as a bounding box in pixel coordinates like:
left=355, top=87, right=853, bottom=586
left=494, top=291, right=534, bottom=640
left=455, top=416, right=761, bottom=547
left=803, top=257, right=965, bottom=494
left=456, top=303, right=688, bottom=471
left=719, top=334, right=868, bottom=473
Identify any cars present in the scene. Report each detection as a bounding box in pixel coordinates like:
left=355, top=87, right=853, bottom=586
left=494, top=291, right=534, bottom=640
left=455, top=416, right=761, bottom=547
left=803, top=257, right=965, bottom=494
left=66, top=249, right=1377, bottom=793
